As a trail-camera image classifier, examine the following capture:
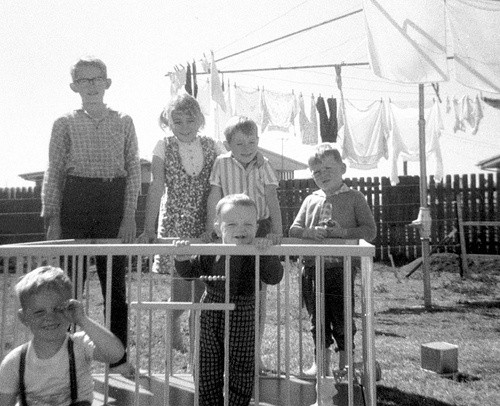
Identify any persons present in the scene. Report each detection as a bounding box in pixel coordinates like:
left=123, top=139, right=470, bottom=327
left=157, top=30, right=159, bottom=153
left=200, top=116, right=284, bottom=375
left=171, top=193, right=284, bottom=406
left=288, top=143, right=378, bottom=382
left=136, top=93, right=265, bottom=354
left=39, top=56, right=146, bottom=380
left=0, top=265, right=125, bottom=406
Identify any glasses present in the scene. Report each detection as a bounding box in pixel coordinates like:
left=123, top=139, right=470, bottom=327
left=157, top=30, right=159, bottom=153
left=74, top=76, right=107, bottom=87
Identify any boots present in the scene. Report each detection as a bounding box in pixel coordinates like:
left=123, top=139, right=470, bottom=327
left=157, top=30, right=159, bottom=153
left=302, top=348, right=331, bottom=380
left=333, top=350, right=355, bottom=372
